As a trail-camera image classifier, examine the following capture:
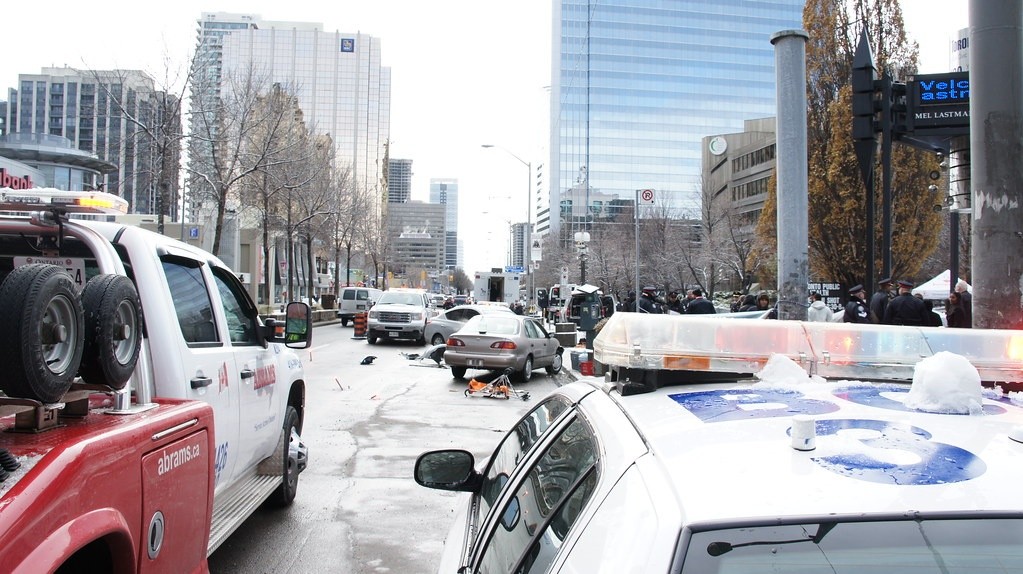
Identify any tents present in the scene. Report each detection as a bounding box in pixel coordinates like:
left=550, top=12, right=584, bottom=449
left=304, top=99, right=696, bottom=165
left=911, top=268, right=971, bottom=299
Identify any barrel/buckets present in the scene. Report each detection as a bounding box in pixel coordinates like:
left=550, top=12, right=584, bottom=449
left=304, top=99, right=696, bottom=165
left=580, top=361, right=593, bottom=375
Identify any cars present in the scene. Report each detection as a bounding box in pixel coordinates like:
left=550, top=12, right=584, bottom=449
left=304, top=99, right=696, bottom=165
left=560, top=291, right=614, bottom=336
left=366, top=288, right=432, bottom=344
left=425, top=293, right=471, bottom=306
left=410, top=310, right=1021, bottom=573
left=549, top=283, right=580, bottom=323
left=424, top=305, right=516, bottom=347
left=443, top=313, right=564, bottom=384
left=337, top=286, right=383, bottom=326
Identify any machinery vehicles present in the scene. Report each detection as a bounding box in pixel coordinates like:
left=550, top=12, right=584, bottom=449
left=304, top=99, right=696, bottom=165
left=0, top=186, right=312, bottom=574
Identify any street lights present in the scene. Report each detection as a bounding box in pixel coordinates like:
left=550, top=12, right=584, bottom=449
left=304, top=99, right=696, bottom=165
left=482, top=144, right=533, bottom=314
left=574, top=231, right=592, bottom=285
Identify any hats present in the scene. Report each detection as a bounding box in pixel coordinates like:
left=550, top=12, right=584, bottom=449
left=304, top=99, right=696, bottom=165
left=643, top=287, right=656, bottom=292
left=849, top=285, right=866, bottom=293
left=896, top=281, right=913, bottom=289
left=878, top=278, right=892, bottom=286
left=668, top=292, right=678, bottom=295
left=692, top=289, right=702, bottom=296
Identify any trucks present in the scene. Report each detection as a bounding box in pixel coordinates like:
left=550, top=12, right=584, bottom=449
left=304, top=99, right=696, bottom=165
left=472, top=268, right=519, bottom=311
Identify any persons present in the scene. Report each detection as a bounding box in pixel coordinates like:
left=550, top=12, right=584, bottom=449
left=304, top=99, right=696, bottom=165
left=443, top=297, right=523, bottom=315
left=616, top=278, right=972, bottom=329
